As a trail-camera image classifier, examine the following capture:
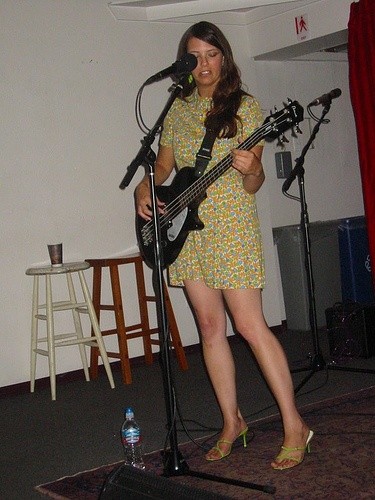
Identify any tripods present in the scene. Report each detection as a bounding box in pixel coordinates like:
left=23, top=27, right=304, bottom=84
left=120, top=75, right=277, bottom=495
left=283, top=103, right=375, bottom=394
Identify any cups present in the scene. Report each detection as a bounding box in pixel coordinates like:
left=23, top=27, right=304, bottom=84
left=47, top=242, right=63, bottom=267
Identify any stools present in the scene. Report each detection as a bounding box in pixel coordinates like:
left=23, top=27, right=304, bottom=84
left=26, top=262, right=115, bottom=401
left=85, top=252, right=189, bottom=384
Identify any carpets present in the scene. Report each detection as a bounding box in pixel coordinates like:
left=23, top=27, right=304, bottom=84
left=32, top=385, right=375, bottom=500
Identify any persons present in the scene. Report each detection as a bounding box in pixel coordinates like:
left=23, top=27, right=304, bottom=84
left=134, top=21, right=314, bottom=469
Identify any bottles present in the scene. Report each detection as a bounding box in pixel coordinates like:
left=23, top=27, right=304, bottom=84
left=122, top=407, right=145, bottom=471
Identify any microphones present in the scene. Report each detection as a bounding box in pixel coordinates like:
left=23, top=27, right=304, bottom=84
left=146, top=53, right=198, bottom=85
left=309, top=88, right=342, bottom=107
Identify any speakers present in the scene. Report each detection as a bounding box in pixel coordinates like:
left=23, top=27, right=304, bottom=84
left=325, top=302, right=375, bottom=359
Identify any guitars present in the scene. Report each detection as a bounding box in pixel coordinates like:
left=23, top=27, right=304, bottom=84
left=133, top=97, right=305, bottom=271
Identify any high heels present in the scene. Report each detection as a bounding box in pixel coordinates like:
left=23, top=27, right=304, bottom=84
left=273, top=431, right=313, bottom=469
left=206, top=427, right=248, bottom=461
